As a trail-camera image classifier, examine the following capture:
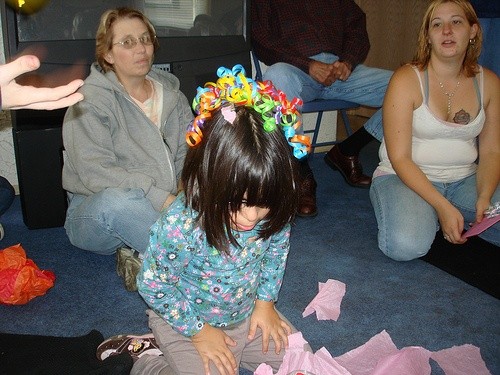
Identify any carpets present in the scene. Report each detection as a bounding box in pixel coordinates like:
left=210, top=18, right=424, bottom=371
left=0, top=139, right=500, bottom=375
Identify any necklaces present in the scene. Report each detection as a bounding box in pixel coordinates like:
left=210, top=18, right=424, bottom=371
left=436, top=81, right=464, bottom=114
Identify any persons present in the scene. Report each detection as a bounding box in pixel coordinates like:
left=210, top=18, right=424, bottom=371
left=369, top=0, right=500, bottom=262
left=0, top=53, right=85, bottom=111
left=247, top=0, right=395, bottom=218
left=96, top=65, right=313, bottom=375
left=61, top=9, right=195, bottom=292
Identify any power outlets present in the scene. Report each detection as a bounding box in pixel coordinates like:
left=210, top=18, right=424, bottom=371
left=152, top=63, right=172, bottom=73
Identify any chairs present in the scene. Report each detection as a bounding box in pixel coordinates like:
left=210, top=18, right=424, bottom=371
left=253, top=48, right=354, bottom=160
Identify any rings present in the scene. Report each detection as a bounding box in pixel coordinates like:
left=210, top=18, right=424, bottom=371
left=443, top=236, right=449, bottom=240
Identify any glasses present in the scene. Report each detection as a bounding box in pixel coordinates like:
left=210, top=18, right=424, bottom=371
left=112, top=32, right=156, bottom=49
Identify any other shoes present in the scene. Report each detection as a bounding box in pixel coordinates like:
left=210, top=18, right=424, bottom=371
left=96, top=333, right=163, bottom=363
left=116, top=247, right=144, bottom=292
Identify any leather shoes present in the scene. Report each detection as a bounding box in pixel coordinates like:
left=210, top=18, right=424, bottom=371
left=323, top=143, right=372, bottom=189
left=295, top=171, right=319, bottom=218
